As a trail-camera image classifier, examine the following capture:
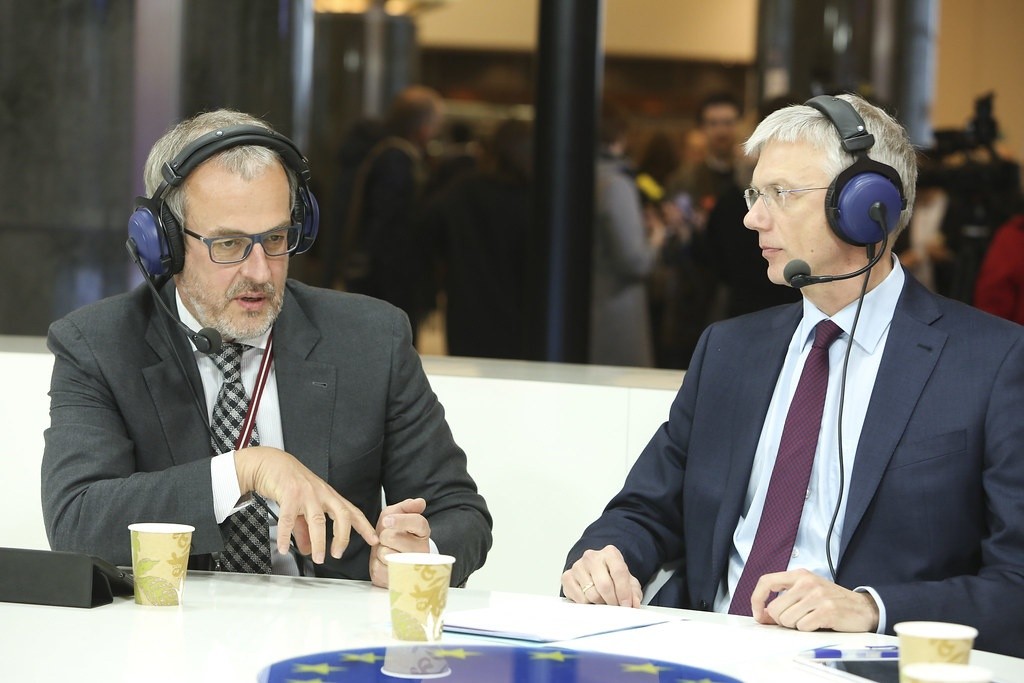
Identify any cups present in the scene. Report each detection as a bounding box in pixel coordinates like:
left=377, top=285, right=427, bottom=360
left=893, top=620, right=994, bottom=683
left=382, top=551, right=456, bottom=644
left=129, top=521, right=194, bottom=608
left=381, top=645, right=452, bottom=679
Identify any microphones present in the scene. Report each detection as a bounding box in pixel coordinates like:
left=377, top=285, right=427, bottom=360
left=782, top=223, right=888, bottom=288
left=135, top=263, right=222, bottom=355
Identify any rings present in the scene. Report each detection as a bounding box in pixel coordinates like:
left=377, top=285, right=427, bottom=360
left=583, top=582, right=595, bottom=592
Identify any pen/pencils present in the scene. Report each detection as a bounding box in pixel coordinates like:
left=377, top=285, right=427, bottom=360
left=798, top=644, right=898, bottom=659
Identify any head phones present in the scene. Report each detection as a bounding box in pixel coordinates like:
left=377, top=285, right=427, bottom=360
left=804, top=94, right=907, bottom=246
left=125, top=124, right=321, bottom=279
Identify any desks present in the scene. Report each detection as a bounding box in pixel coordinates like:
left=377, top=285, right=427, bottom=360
left=0, top=567, right=1024, bottom=683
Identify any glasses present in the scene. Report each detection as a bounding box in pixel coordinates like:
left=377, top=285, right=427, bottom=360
left=744, top=184, right=830, bottom=214
left=180, top=216, right=302, bottom=265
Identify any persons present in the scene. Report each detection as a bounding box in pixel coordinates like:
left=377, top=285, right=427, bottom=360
left=555, top=94, right=1024, bottom=655
left=41, top=109, right=494, bottom=590
left=327, top=84, right=1024, bottom=370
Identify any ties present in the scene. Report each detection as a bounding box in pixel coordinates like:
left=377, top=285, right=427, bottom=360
left=205, top=342, right=273, bottom=575
left=728, top=321, right=844, bottom=616
